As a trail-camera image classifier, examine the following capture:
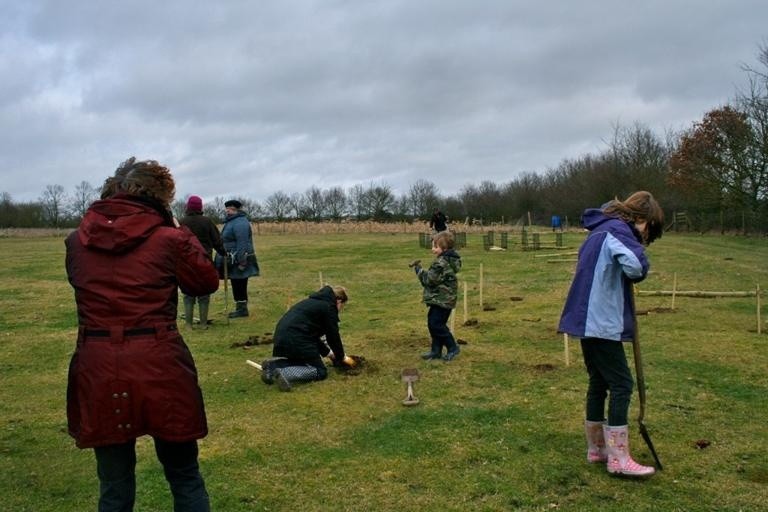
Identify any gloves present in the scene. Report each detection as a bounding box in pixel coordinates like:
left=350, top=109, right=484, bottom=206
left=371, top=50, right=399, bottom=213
left=344, top=355, right=357, bottom=369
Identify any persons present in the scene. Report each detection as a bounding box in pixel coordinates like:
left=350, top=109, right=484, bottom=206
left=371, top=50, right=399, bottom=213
left=557, top=187, right=666, bottom=476
left=261, top=285, right=356, bottom=392
left=212, top=200, right=260, bottom=319
left=428, top=206, right=448, bottom=233
left=550, top=215, right=564, bottom=233
left=177, top=194, right=231, bottom=333
left=406, top=232, right=465, bottom=362
left=62, top=156, right=222, bottom=512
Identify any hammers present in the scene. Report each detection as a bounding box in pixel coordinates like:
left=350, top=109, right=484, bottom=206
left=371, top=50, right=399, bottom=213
left=407, top=258, right=422, bottom=269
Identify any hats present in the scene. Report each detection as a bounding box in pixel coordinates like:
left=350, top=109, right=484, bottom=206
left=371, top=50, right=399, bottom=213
left=225, top=200, right=242, bottom=208
left=187, top=196, right=202, bottom=213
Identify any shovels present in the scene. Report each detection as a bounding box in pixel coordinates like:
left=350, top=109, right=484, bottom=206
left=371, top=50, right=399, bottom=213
left=630, top=285, right=663, bottom=470
left=401, top=368, right=420, bottom=405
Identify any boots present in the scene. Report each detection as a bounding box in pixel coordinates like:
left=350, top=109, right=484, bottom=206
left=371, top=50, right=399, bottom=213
left=584, top=420, right=607, bottom=463
left=185, top=304, right=193, bottom=330
left=229, top=300, right=248, bottom=318
left=200, top=303, right=209, bottom=330
left=602, top=425, right=657, bottom=478
left=443, top=336, right=460, bottom=360
left=275, top=366, right=317, bottom=394
left=421, top=343, right=441, bottom=360
left=261, top=359, right=292, bottom=384
